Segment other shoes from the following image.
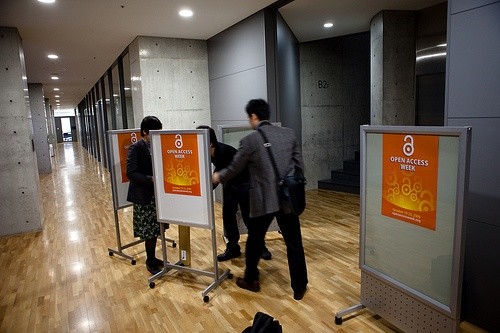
[155,257,169,267]
[145,259,162,275]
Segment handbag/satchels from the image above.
[276,175,307,217]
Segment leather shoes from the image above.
[236,277,258,291]
[262,246,272,260]
[294,288,307,300]
[217,249,241,262]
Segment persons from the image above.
[211,99,309,300]
[126,116,169,276]
[196,125,271,262]
